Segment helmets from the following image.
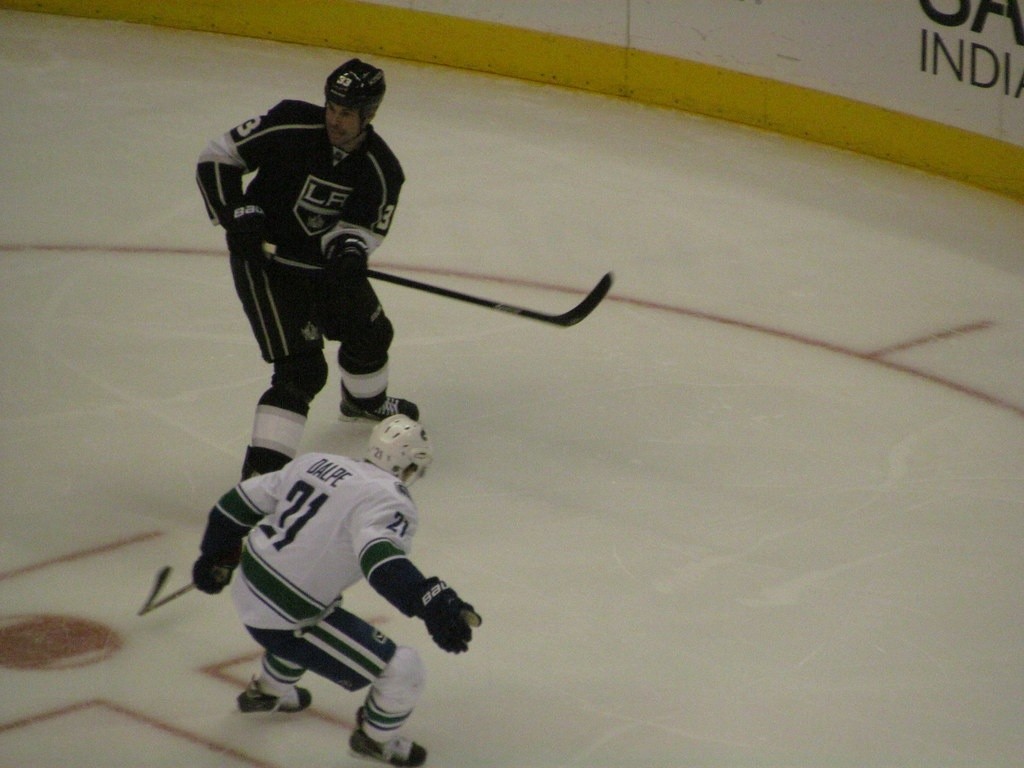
[366,414,434,487]
[325,59,386,108]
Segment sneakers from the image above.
[338,377,419,423]
[349,706,426,768]
[236,672,312,720]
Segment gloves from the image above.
[318,235,369,293]
[412,576,481,654]
[193,539,242,594]
[220,194,277,265]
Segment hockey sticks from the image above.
[139,566,198,617]
[271,247,613,328]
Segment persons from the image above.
[192,415,481,767]
[197,57,419,480]
